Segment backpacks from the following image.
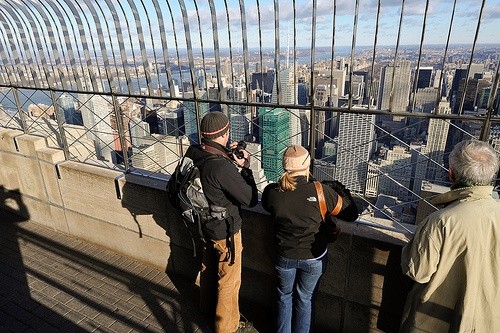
[173,154,232,225]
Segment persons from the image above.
[400,140,500,333]
[261,144,358,333]
[185,110,258,333]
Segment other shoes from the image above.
[236,320,253,333]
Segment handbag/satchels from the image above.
[313,180,342,243]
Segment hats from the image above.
[283,145,311,171]
[201,112,230,138]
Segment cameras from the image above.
[229,141,246,160]
